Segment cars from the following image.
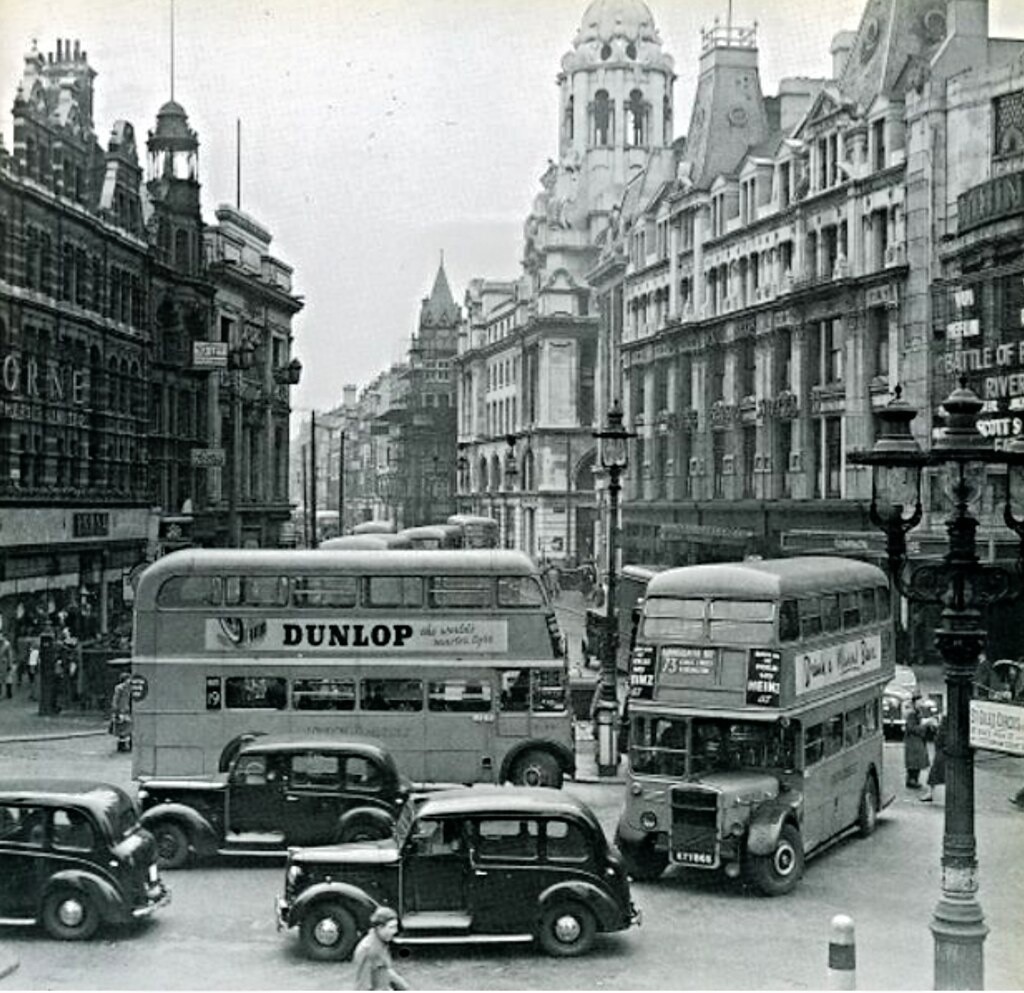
[883,685,938,741]
[130,735,464,869]
[0,776,168,941]
[274,785,642,957]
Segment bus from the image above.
[318,515,501,552]
[614,556,897,896]
[130,550,576,816]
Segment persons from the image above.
[350,907,408,991]
[113,673,131,753]
[904,695,949,803]
[0,597,99,700]
[543,557,604,608]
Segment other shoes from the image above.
[906,782,923,788]
[921,795,932,802]
[1009,796,1024,807]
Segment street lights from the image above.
[845,383,1024,991]
[593,399,639,778]
